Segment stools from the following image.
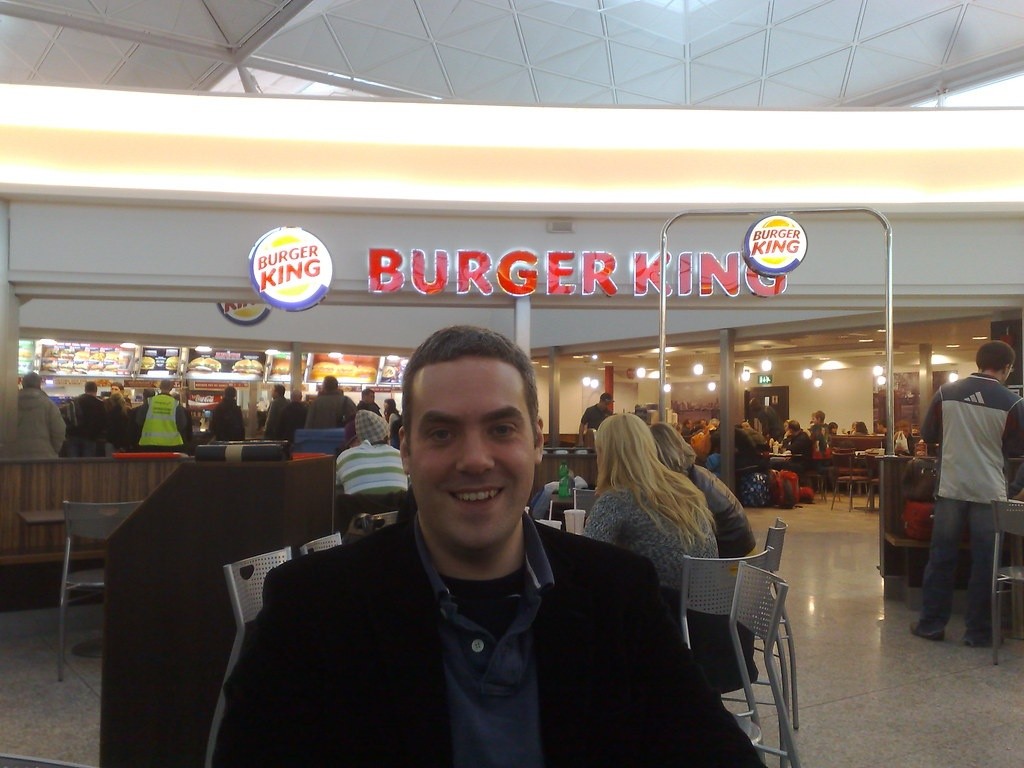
[805,472,827,502]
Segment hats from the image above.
[343,414,356,448]
[355,410,389,444]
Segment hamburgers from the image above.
[166,356,178,371]
[312,362,376,381]
[189,358,263,374]
[141,357,155,370]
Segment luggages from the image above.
[739,467,800,508]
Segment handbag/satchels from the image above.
[899,457,971,542]
[799,486,814,504]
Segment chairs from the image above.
[986,499,1024,665]
[59,450,800,768]
[830,448,882,512]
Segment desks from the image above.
[768,453,802,456]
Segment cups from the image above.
[564,509,586,535]
[877,449,885,456]
[536,519,562,530]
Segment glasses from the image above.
[1008,366,1016,373]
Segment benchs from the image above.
[16,509,118,553]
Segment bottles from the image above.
[559,460,575,498]
[769,437,783,455]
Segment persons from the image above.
[807,410,839,492]
[213,321,772,768]
[384,397,399,421]
[780,419,811,477]
[258,396,266,425]
[679,416,722,477]
[279,389,308,457]
[264,384,290,440]
[876,417,887,450]
[63,381,133,458]
[579,394,616,448]
[648,418,758,662]
[910,341,1024,648]
[209,387,245,441]
[846,421,869,435]
[356,389,383,418]
[16,372,66,458]
[584,412,731,674]
[896,419,921,457]
[734,417,776,473]
[304,375,358,431]
[131,380,192,453]
[334,412,409,494]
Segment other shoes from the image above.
[962,628,994,647]
[910,621,944,640]
